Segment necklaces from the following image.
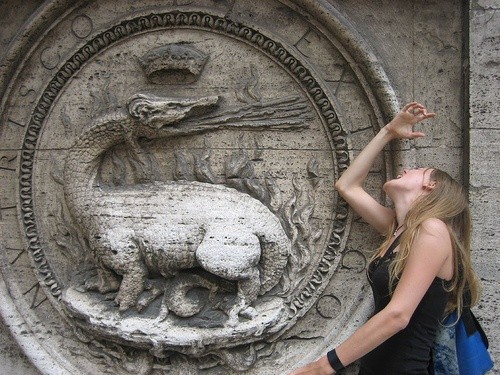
[392,223,407,237]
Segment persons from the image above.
[288,100,480,375]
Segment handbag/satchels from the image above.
[428,306,493,374]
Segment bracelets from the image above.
[326,349,347,375]
